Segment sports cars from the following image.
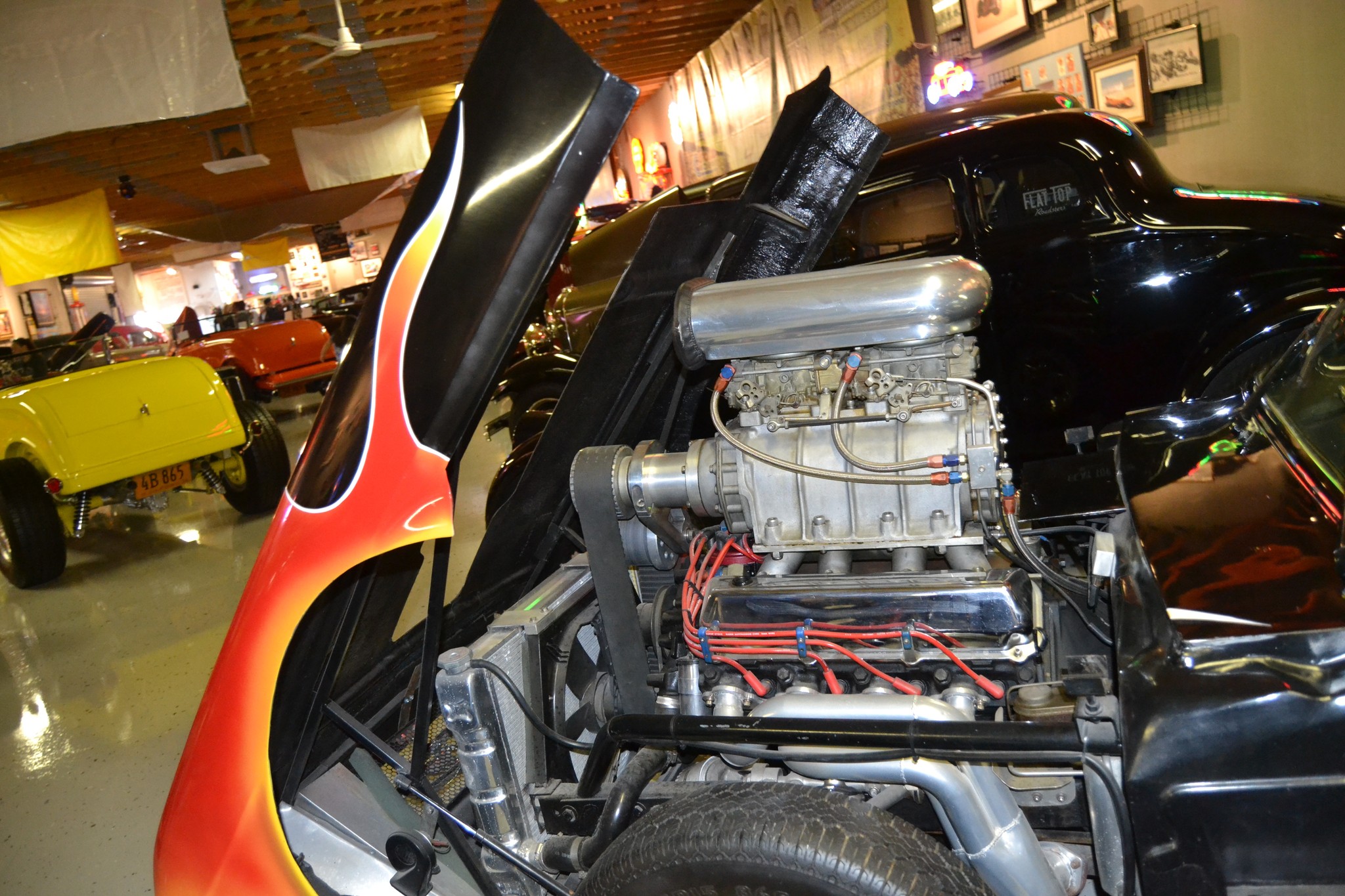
[25,324,170,372]
[93,307,341,403]
[152,0,1345,896]
[311,281,381,349]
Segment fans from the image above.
[288,0,438,71]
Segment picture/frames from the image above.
[1141,23,1208,96]
[961,0,1034,57]
[1085,1,1121,51]
[930,0,964,37]
[1085,43,1157,127]
[1018,42,1092,109]
[1028,0,1060,16]
[982,81,1026,100]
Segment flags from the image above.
[0,188,124,289]
[0,0,247,150]
[240,237,290,273]
[291,105,431,193]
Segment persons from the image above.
[2,335,48,388]
[211,286,333,333]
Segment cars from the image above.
[0,328,289,587]
